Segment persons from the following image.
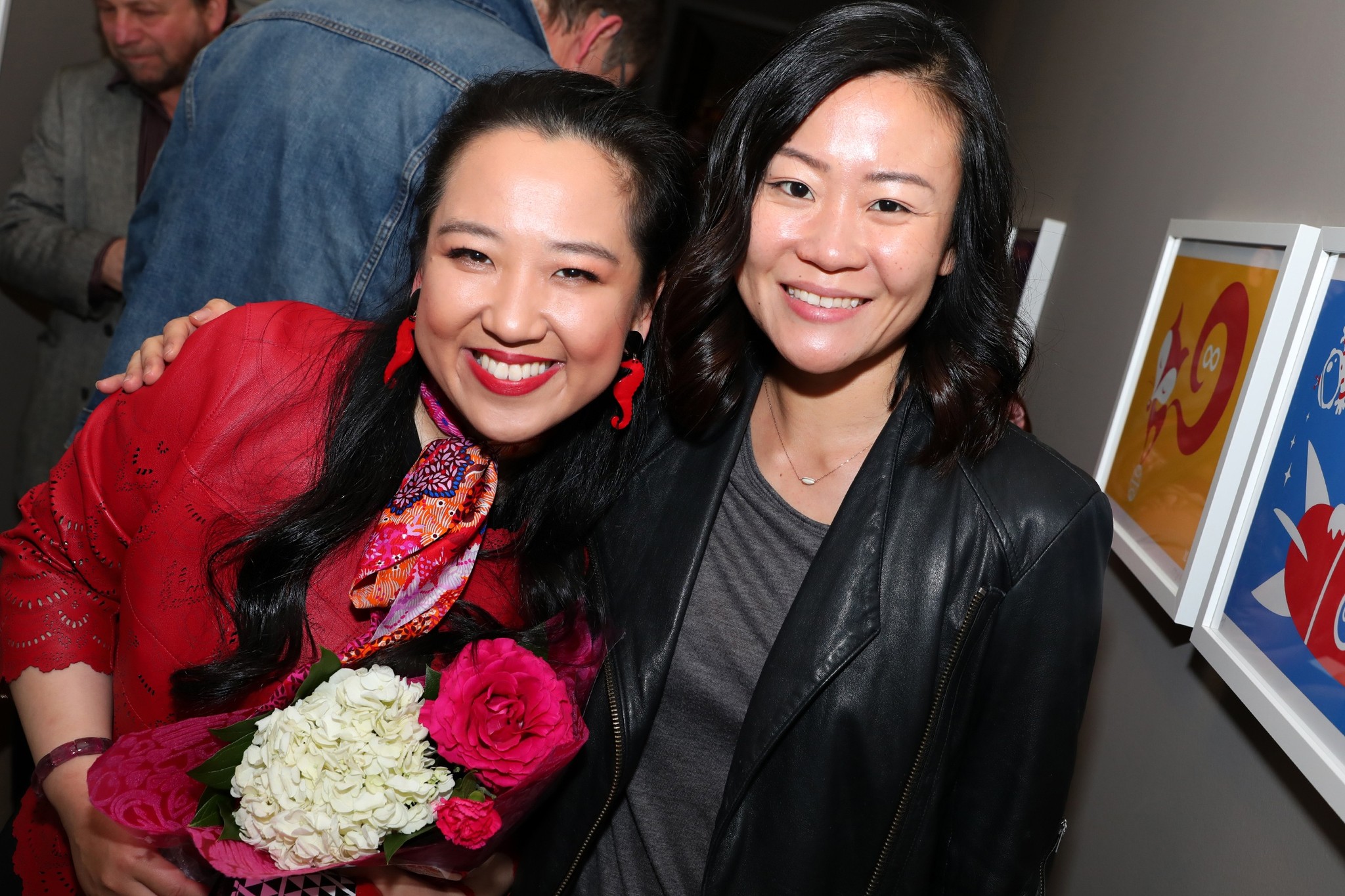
[0,0,230,533]
[95,0,1112,895]
[0,68,696,896]
[75,0,673,429]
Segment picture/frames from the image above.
[1091,216,1323,629]
[1187,224,1345,828]
[1002,215,1067,375]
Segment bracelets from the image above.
[30,737,113,800]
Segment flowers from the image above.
[84,608,607,880]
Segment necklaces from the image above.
[764,376,873,486]
